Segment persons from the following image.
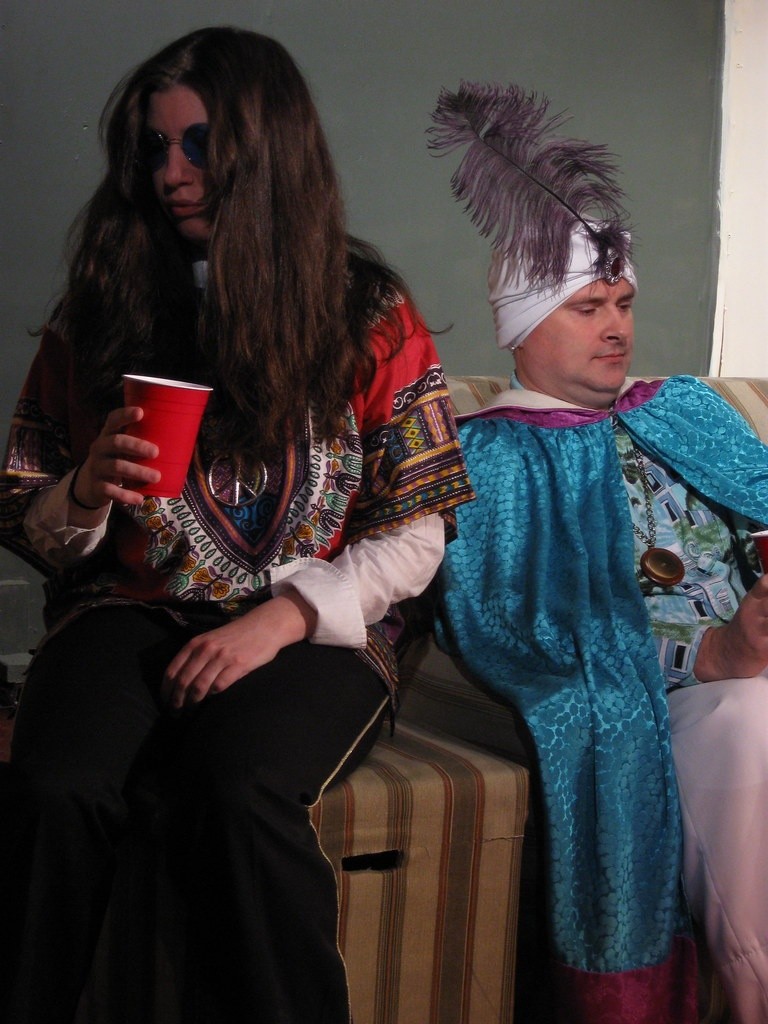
[432,206,767,1024]
[0,27,476,1024]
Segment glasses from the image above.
[133,120,222,175]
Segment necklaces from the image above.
[632,444,685,584]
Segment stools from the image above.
[0,711,530,1024]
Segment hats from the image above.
[422,78,639,353]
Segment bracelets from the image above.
[70,461,110,510]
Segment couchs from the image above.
[397,375,767,1024]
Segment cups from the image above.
[751,530,768,574]
[121,374,213,503]
